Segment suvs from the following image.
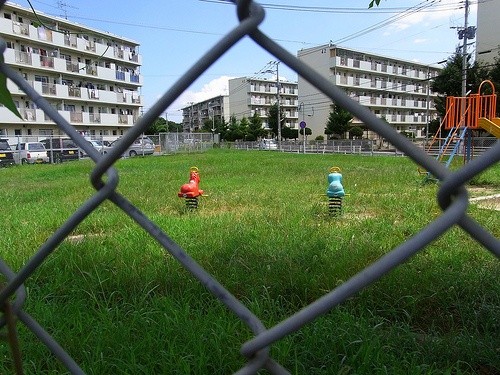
[0,138,16,167]
[39,138,79,163]
[110,135,155,157]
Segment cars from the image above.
[81,140,113,158]
[256,139,278,150]
[10,143,49,166]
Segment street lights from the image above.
[299,101,305,154]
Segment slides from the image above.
[478,117,500,139]
